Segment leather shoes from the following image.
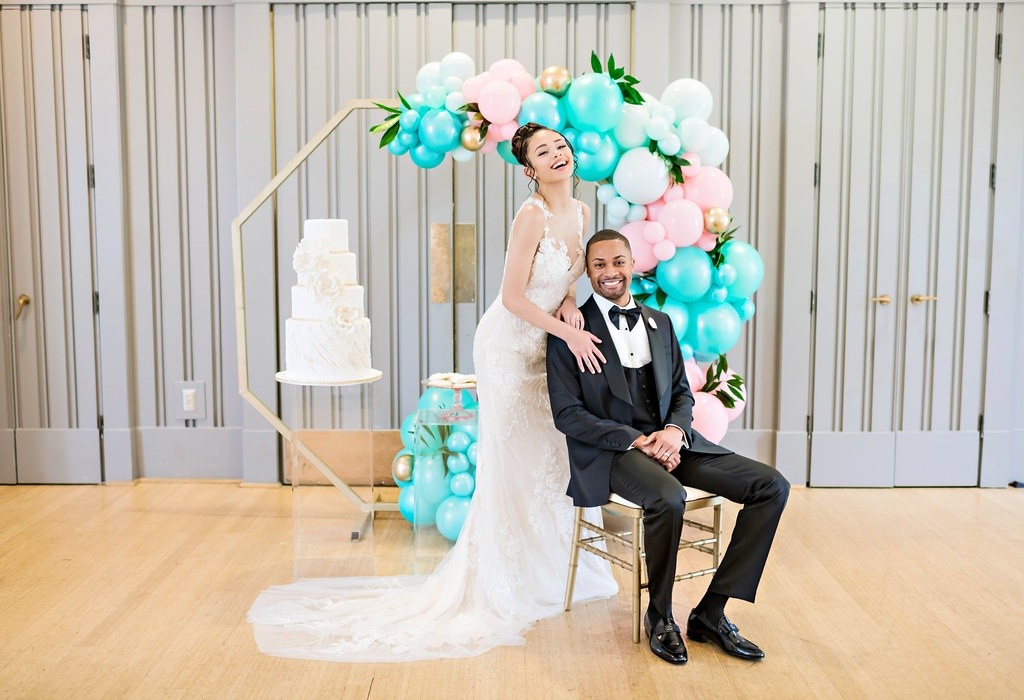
[687,607,764,659]
[644,609,688,663]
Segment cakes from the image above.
[285,219,371,382]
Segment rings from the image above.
[576,320,579,321]
[665,454,669,457]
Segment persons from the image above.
[454,121,620,614]
[546,229,792,664]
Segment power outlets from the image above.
[182,389,196,411]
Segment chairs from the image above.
[563,482,727,643]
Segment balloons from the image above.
[391,387,479,541]
[625,153,763,444]
[540,67,572,97]
[479,59,536,155]
[704,207,729,233]
[497,71,627,183]
[597,80,729,227]
[387,52,485,168]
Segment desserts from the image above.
[428,372,475,389]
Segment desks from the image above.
[274,368,384,588]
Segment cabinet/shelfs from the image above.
[411,407,481,572]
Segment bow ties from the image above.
[608,305,642,331]
